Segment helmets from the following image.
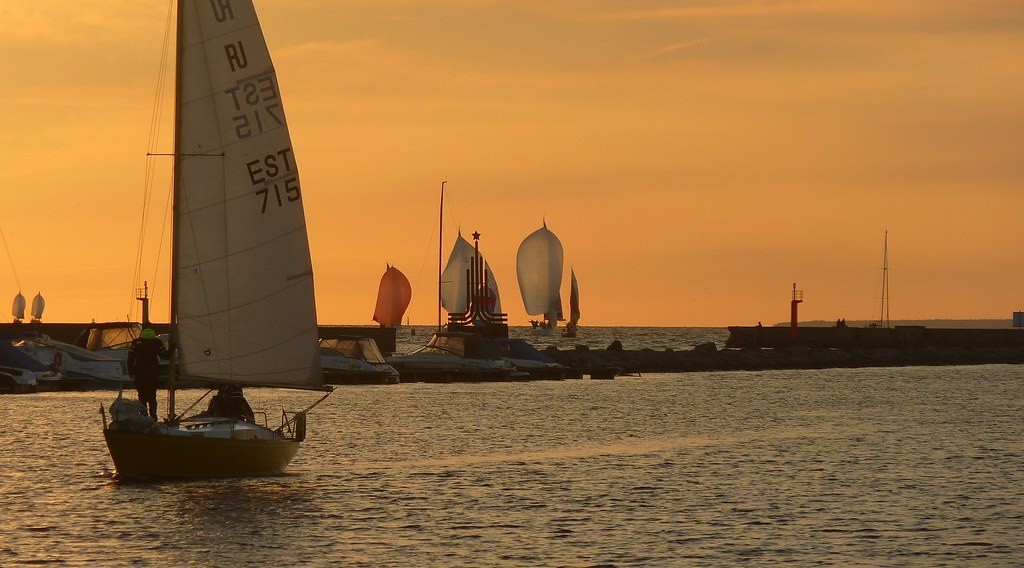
[140,328,155,340]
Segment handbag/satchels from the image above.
[128,347,148,378]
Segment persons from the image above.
[127,328,169,422]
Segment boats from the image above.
[1,321,622,394]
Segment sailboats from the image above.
[423,178,509,347]
[12,290,26,323]
[561,270,580,339]
[516,218,567,337]
[29,290,46,324]
[371,261,412,327]
[99,1,339,482]
